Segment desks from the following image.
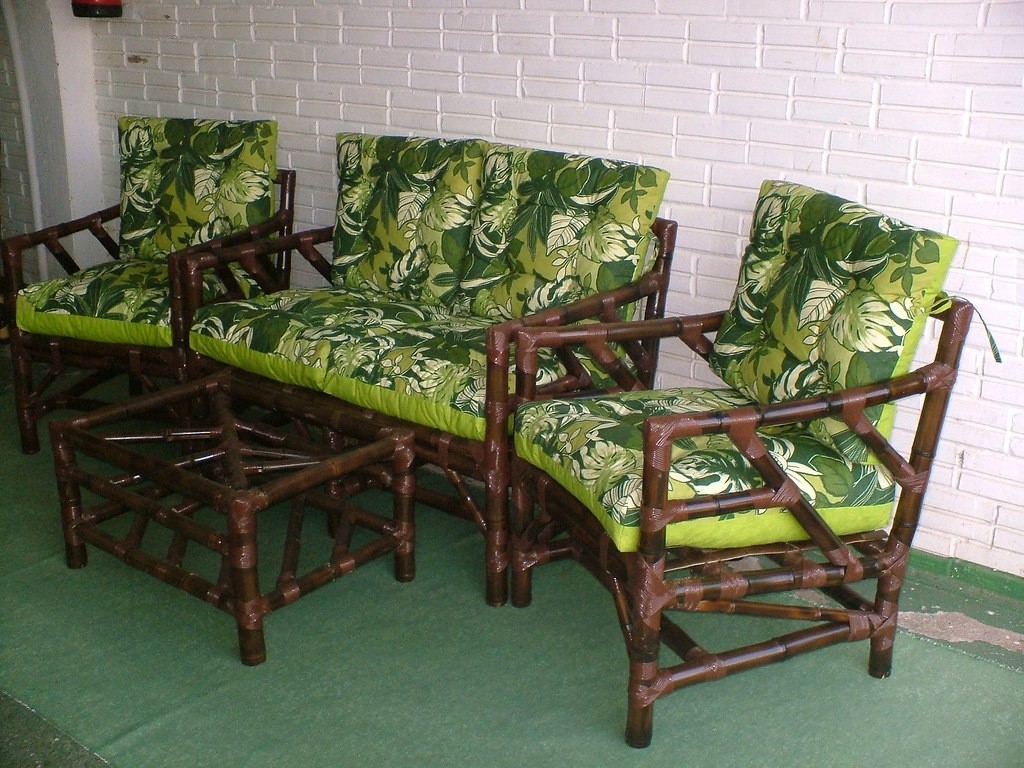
[48,370,416,668]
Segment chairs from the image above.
[0,115,298,482]
[509,179,1005,749]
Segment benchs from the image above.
[178,131,679,608]
